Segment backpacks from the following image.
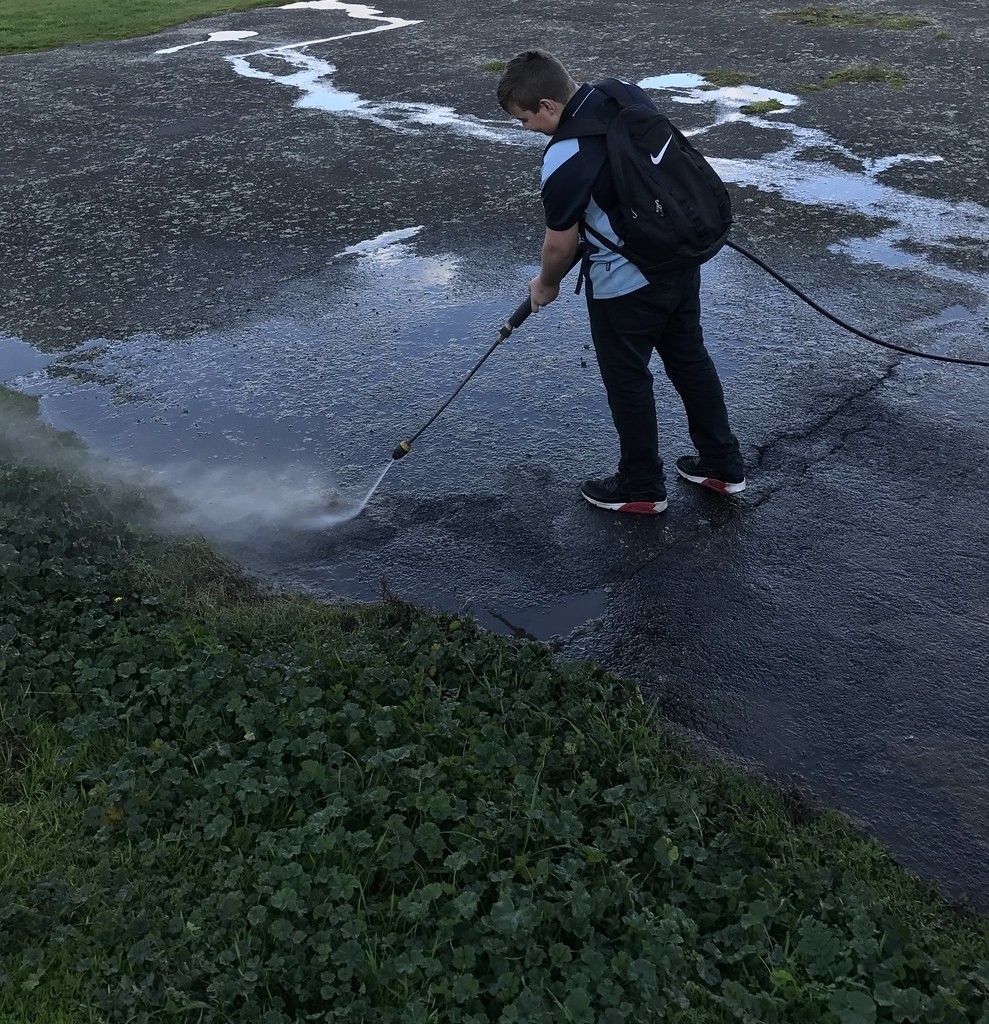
[541,77,732,272]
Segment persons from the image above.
[494,49,748,513]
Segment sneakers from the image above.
[676,455,746,495]
[580,472,668,515]
